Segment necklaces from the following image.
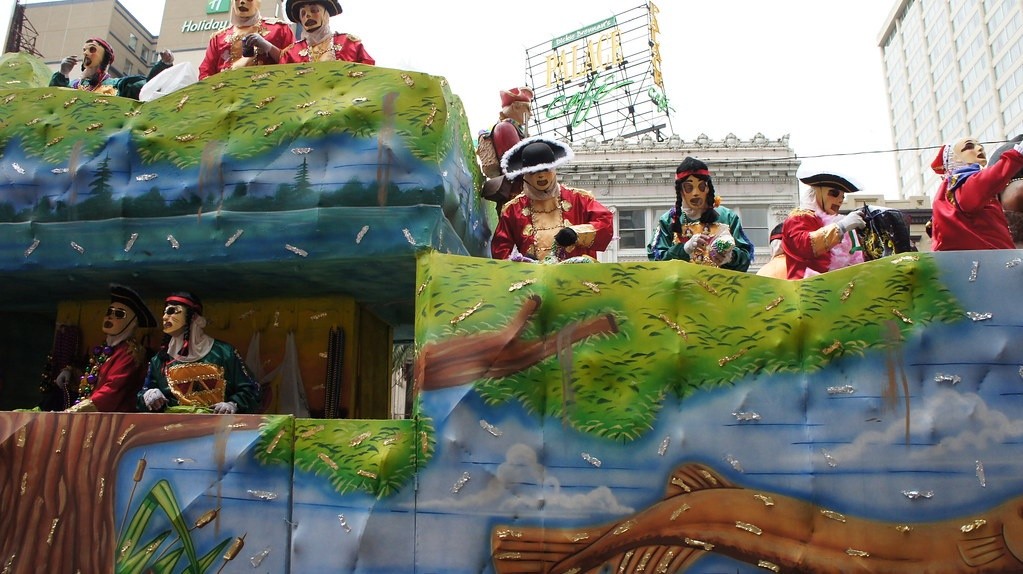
[325,326,344,418]
[75,343,112,404]
[64,384,70,409]
[91,73,108,91]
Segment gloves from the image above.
[840,210,866,232]
[55,366,74,388]
[61,55,78,75]
[555,227,577,248]
[245,33,272,54]
[209,401,238,415]
[142,388,168,411]
[160,49,175,64]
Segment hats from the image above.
[285,0,343,23]
[86,38,115,65]
[932,143,949,175]
[796,157,864,193]
[500,137,574,179]
[109,283,160,328]
[500,86,534,107]
[165,292,204,316]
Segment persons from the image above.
[755,223,785,279]
[647,156,752,272]
[136,291,262,414]
[493,87,534,205]
[492,136,613,261]
[930,135,1023,252]
[280,0,375,65]
[199,0,296,79]
[783,173,867,279]
[49,38,174,99]
[54,284,157,411]
